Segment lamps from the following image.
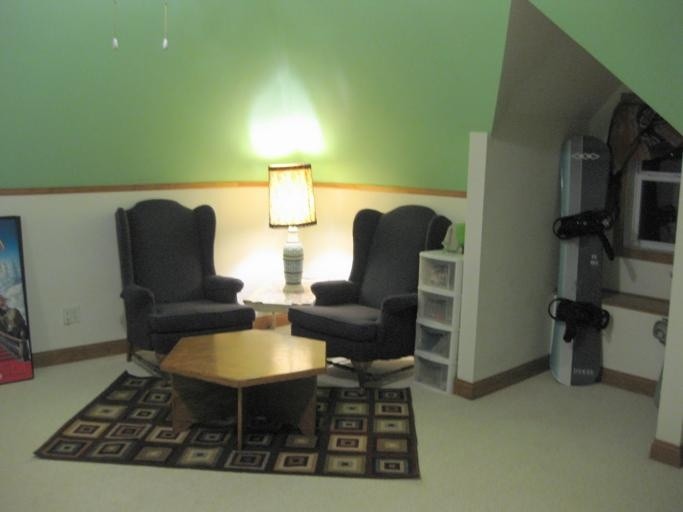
[266,161,319,292]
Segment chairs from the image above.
[288,203,452,398]
[115,198,256,368]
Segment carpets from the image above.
[32,366,425,482]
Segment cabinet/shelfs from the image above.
[413,246,455,397]
